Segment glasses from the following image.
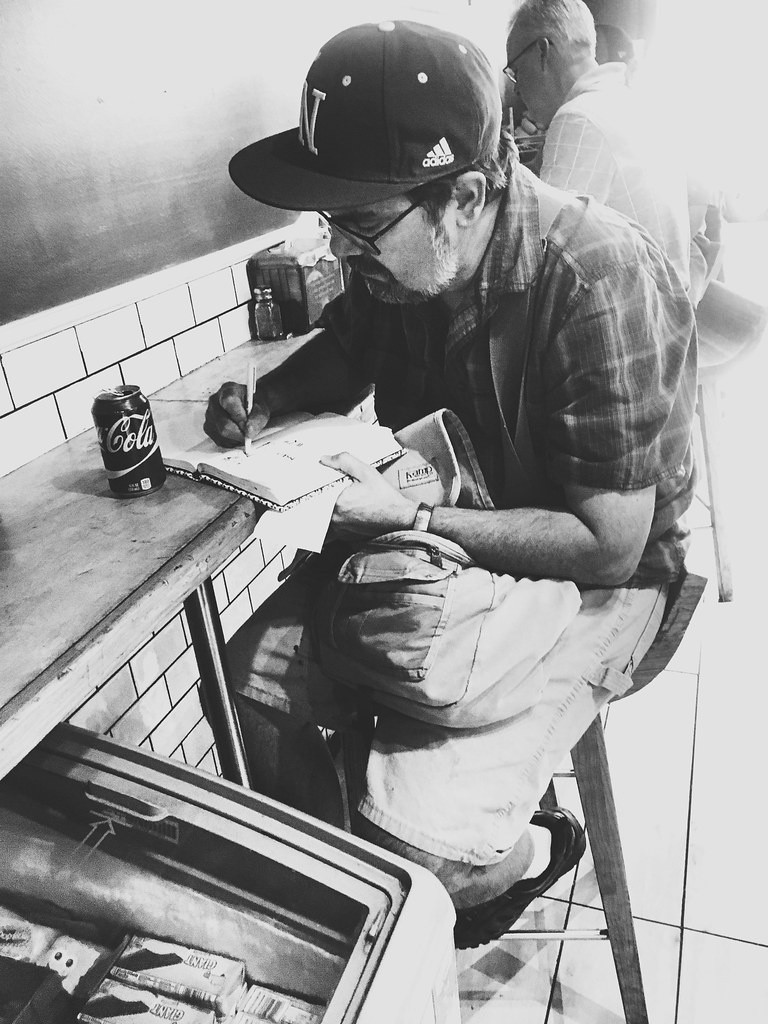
[314,187,449,258]
[502,36,553,84]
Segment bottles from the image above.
[253,285,282,341]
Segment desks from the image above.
[0,328,376,789]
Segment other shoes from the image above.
[454,806,587,951]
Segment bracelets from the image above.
[412,500,434,533]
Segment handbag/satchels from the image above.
[306,526,582,730]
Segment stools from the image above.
[689,281,768,602]
[343,573,708,1024]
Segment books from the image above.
[162,409,409,512]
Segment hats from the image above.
[227,19,502,211]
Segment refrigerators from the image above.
[0,720,460,1024]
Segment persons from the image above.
[499,0,722,315]
[202,22,699,949]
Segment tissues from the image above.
[246,210,345,335]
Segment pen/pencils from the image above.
[244,363,257,457]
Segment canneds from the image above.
[90,385,166,498]
